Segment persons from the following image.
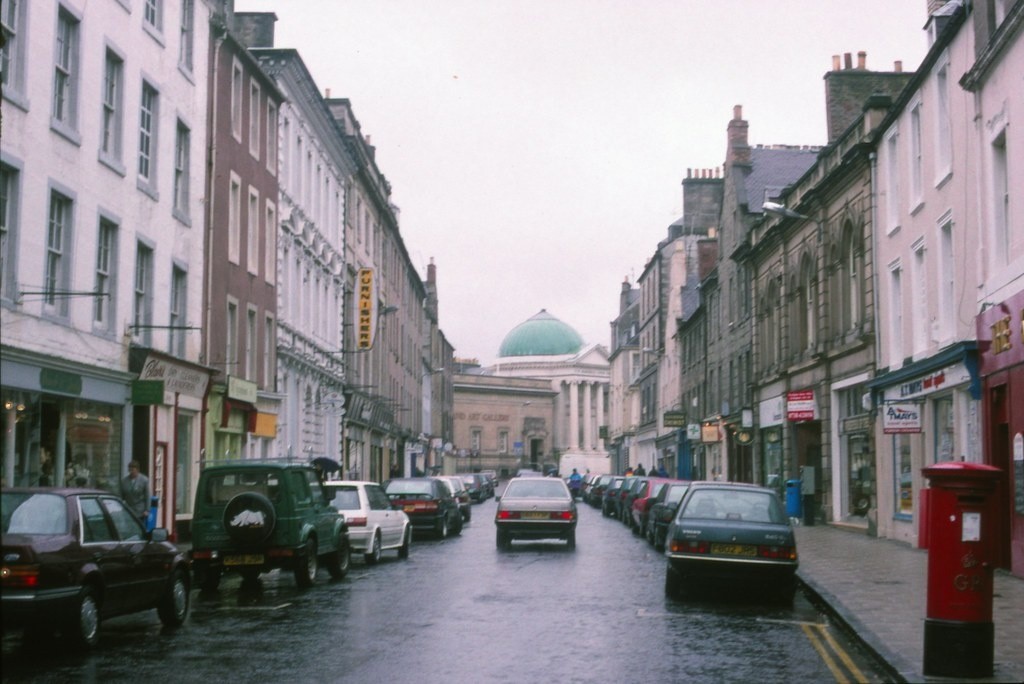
[621,462,669,478]
[39,449,89,489]
[390,463,444,478]
[316,463,327,481]
[120,460,151,528]
[569,469,581,502]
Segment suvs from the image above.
[193,459,352,591]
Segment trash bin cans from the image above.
[784,479,802,518]
[144,496,159,534]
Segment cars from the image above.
[580,473,691,552]
[386,477,463,540]
[444,470,499,521]
[494,476,577,547]
[515,468,545,477]
[1,487,194,654]
[665,480,798,586]
[309,480,413,566]
